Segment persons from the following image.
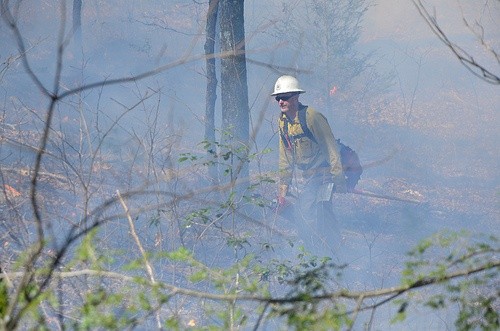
[269,74,361,259]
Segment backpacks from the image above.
[283,105,362,194]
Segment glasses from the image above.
[274,93,297,101]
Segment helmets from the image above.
[270,75,306,96]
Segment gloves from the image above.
[278,185,289,205]
[334,174,347,196]
